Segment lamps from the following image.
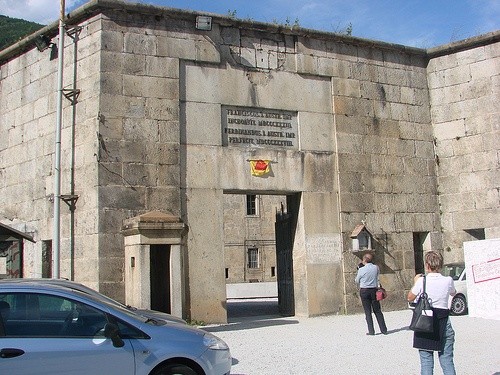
[34,35,51,51]
[195,15,212,31]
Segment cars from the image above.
[0,277,232,375]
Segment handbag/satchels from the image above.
[409,292,434,334]
[376,288,387,301]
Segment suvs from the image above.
[408,262,468,316]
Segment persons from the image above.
[355,254,387,335]
[408,251,456,375]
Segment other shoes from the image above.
[366,333,375,336]
